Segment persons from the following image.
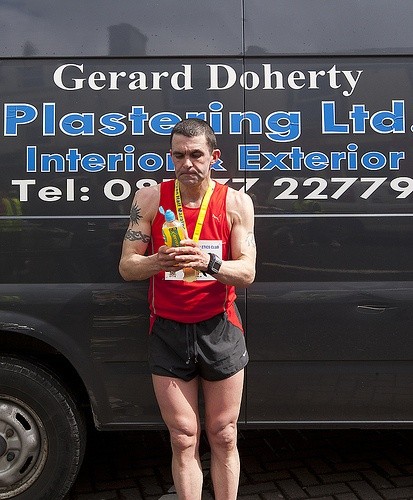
[119,119,256,499]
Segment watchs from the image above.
[200,253,221,277]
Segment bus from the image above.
[0,1,413,500]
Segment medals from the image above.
[181,263,197,284]
